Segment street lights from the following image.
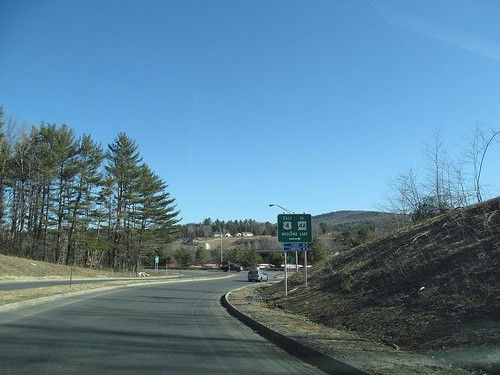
[268,203,298,275]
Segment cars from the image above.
[248,270,269,282]
[222,263,244,272]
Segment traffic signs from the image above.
[278,213,313,243]
